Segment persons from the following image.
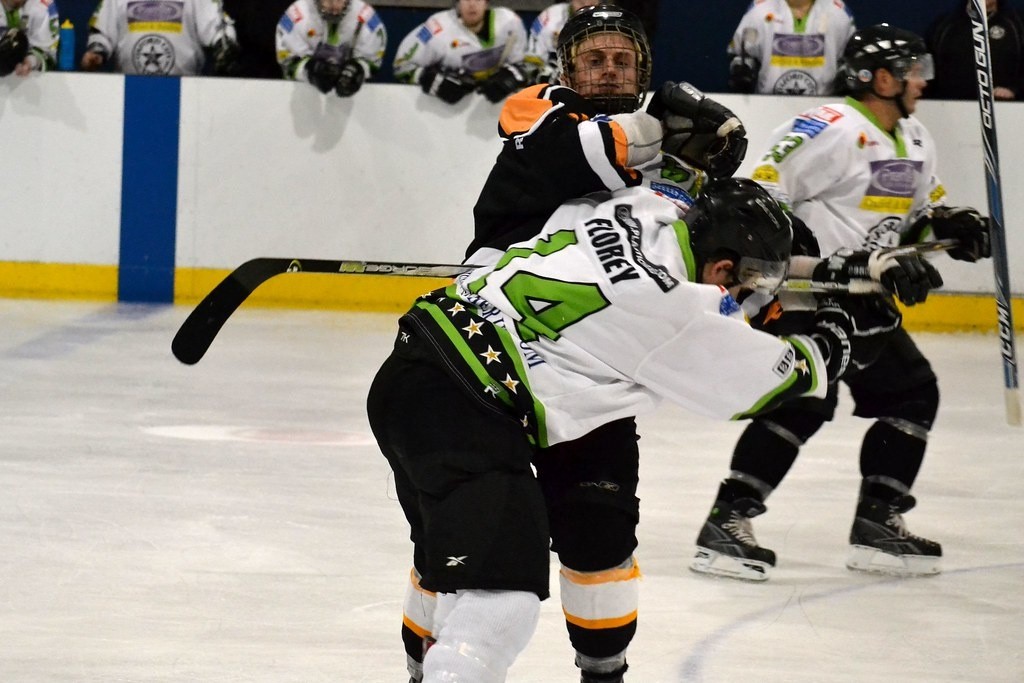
[730,0,860,98]
[684,21,992,583]
[0,0,241,80]
[276,0,387,97]
[367,6,898,682]
[395,0,605,107]
[933,1,1018,100]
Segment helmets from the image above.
[842,24,927,90]
[690,178,793,295]
[557,6,652,73]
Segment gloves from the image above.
[931,206,992,263]
[418,62,478,105]
[309,58,365,97]
[480,61,529,104]
[807,247,944,385]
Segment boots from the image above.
[847,493,944,573]
[689,482,776,582]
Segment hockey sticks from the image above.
[169,256,884,369]
[886,239,962,260]
[970,0,1023,427]
